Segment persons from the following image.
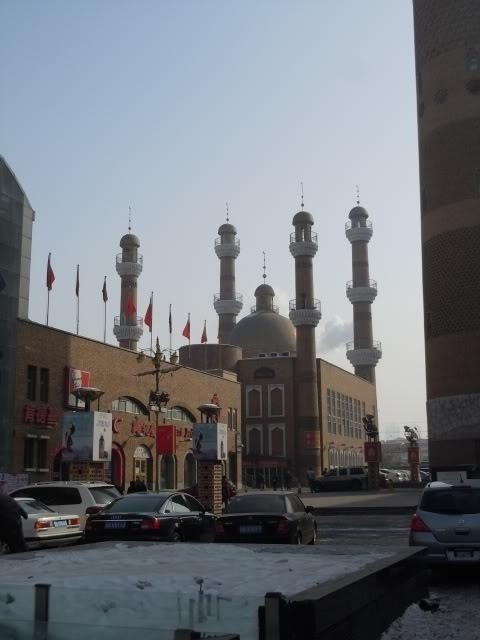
[211,392,220,423]
[136,477,148,492]
[126,481,137,494]
[284,470,293,491]
[222,475,229,510]
[306,467,315,493]
[321,467,328,475]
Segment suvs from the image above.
[406,475,479,567]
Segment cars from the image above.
[6,479,214,546]
[211,490,319,547]
[311,464,431,491]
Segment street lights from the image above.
[136,336,183,491]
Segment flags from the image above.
[102,279,108,303]
[143,298,152,332]
[182,320,189,338]
[200,322,207,343]
[77,266,80,297]
[169,306,172,333]
[47,256,55,291]
